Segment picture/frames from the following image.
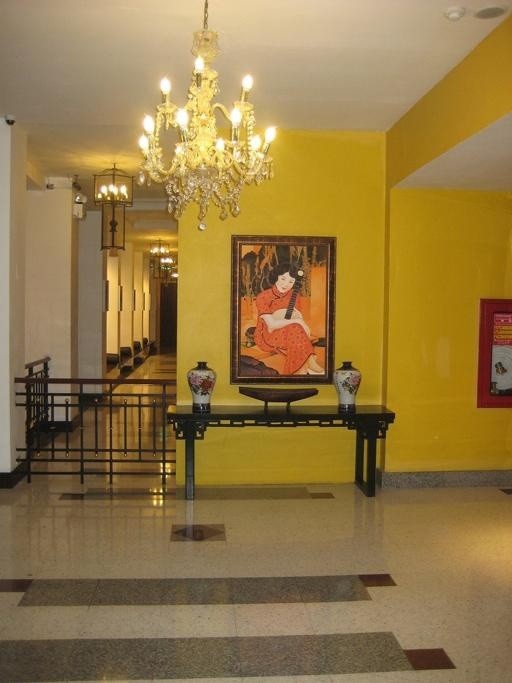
[225,231,335,385]
[476,297,511,409]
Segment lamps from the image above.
[137,1,277,230]
[147,238,178,280]
[93,162,134,258]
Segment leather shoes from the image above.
[308,368,324,375]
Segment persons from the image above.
[254,261,325,375]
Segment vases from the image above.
[186,359,216,411]
[331,360,360,412]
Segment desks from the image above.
[164,403,394,501]
